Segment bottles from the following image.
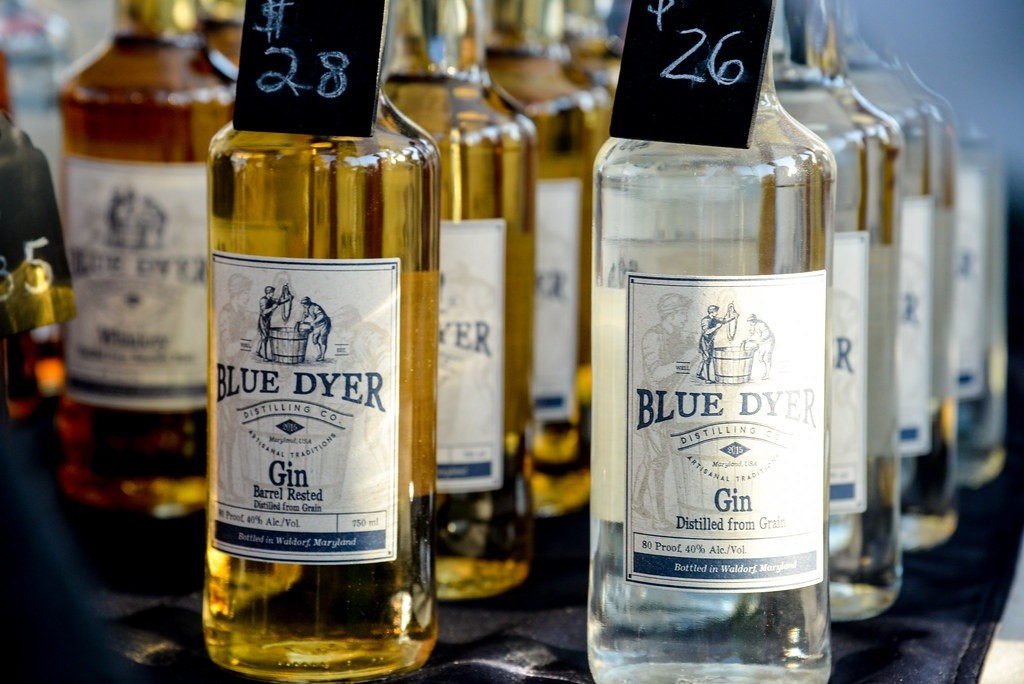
[1,0,1007,684]
